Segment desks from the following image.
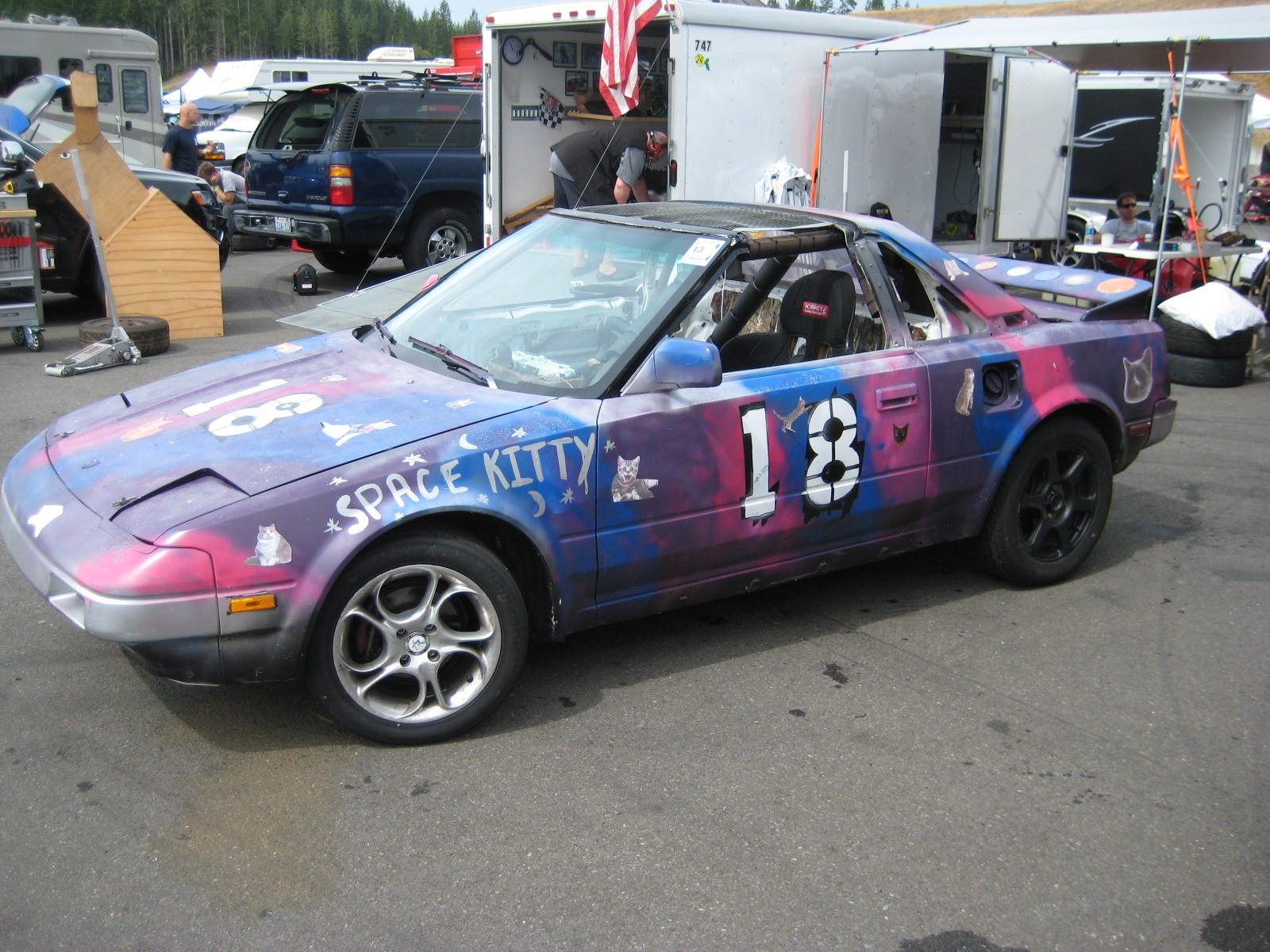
[1074,240,1262,292]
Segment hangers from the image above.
[516,35,554,62]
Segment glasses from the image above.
[1120,202,1136,208]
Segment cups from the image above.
[207,139,214,154]
[1101,233,1114,249]
[1144,234,1153,242]
[1177,243,1191,251]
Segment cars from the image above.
[0,199,1180,748]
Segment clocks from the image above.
[501,35,524,64]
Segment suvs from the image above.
[0,104,231,302]
[194,101,275,178]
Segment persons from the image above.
[162,103,215,177]
[1094,192,1154,279]
[549,128,668,282]
[197,161,247,253]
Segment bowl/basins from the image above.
[1243,238,1255,246]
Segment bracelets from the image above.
[212,186,220,191]
[203,148,206,155]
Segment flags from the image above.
[599,0,664,118]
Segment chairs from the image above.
[719,270,856,372]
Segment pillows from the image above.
[1156,281,1268,340]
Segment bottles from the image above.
[1083,217,1095,247]
[1197,228,1207,242]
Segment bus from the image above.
[1,21,169,171]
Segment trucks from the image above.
[481,0,1257,249]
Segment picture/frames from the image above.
[646,74,666,90]
[660,47,669,73]
[639,46,656,72]
[565,70,588,95]
[592,71,600,93]
[553,41,577,68]
[581,43,603,69]
